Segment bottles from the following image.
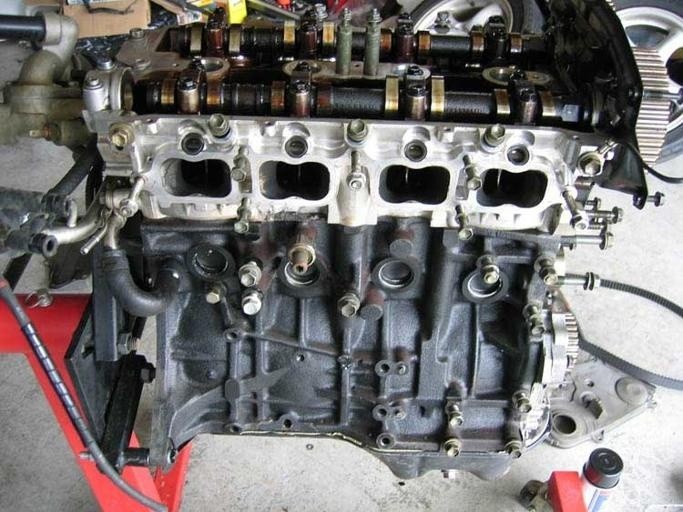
[580,447,624,512]
